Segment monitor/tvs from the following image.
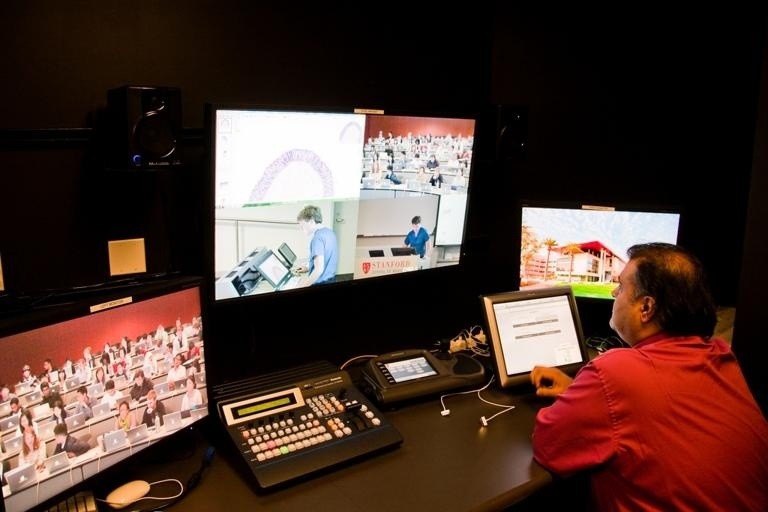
[278,243,296,266]
[0,275,216,512]
[391,247,414,255]
[368,249,384,257]
[254,250,289,289]
[206,102,482,308]
[513,194,683,315]
[479,292,594,394]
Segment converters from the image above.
[450,335,486,351]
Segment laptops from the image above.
[363,138,468,193]
[1,347,204,492]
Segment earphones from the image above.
[480,416,488,427]
[440,409,451,417]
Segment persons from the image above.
[0,310,206,480]
[362,125,473,194]
[403,214,431,260]
[530,240,767,512]
[287,204,339,288]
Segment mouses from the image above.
[104,478,151,511]
